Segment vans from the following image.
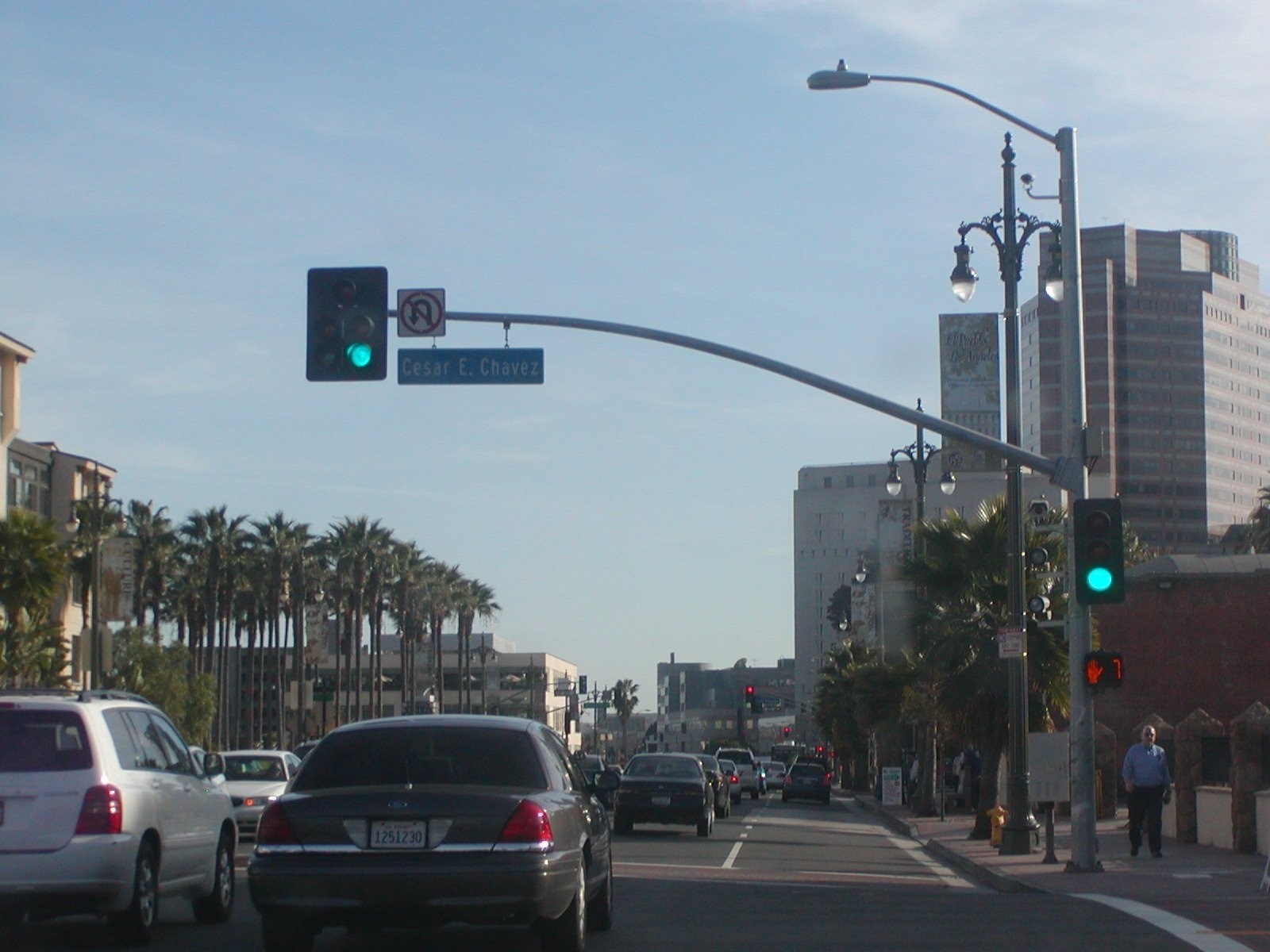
[794,755,828,773]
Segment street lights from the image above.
[278,549,327,745]
[947,129,1064,852]
[396,609,428,716]
[521,653,545,721]
[885,397,957,816]
[65,461,127,690]
[854,550,890,802]
[808,57,1100,873]
[468,632,497,714]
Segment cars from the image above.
[572,754,604,785]
[759,761,788,795]
[187,740,321,839]
[245,712,622,952]
[719,759,744,806]
[613,751,718,837]
[655,753,733,820]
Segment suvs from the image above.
[715,747,761,800]
[782,764,831,805]
[0,686,239,950]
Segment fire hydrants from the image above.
[986,804,1008,848]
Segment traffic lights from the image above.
[745,684,754,703]
[784,727,789,737]
[818,747,823,759]
[1085,652,1123,687]
[306,265,389,382]
[1073,497,1125,605]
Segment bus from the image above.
[771,741,807,772]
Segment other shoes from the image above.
[1148,849,1162,858]
[1130,839,1142,857]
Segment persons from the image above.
[1121,726,1171,857]
[906,748,965,807]
